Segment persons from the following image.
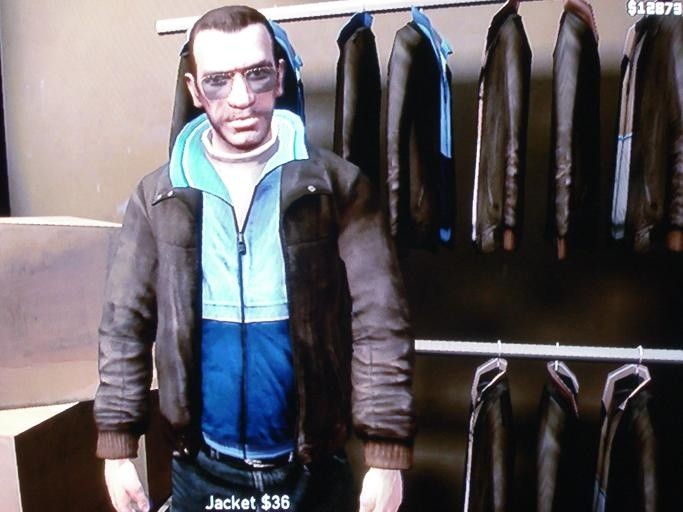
[93,5,415,512]
